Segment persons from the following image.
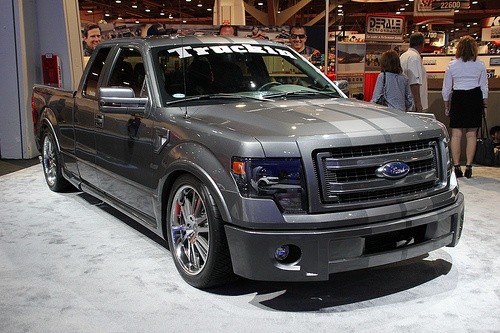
[442,35,489,178]
[399,32,429,113]
[81,22,323,64]
[367,49,413,112]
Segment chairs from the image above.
[186,59,213,97]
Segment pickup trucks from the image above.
[28,32,466,292]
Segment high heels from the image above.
[465,165,472,178]
[454,165,463,178]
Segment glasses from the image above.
[290,33,305,39]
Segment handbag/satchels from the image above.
[475,140,495,163]
[376,95,388,107]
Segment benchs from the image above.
[118,61,145,86]
